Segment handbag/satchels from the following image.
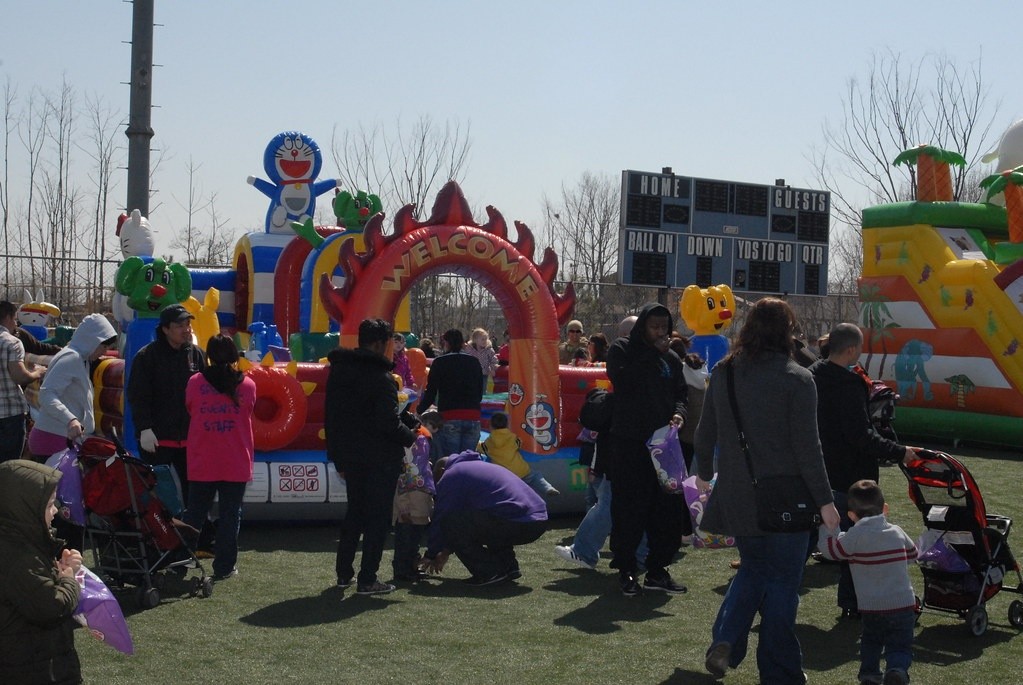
[579,390,614,432]
[681,471,738,548]
[645,423,689,495]
[43,446,88,527]
[917,529,980,591]
[71,564,135,656]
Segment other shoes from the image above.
[729,560,740,568]
[459,567,523,586]
[217,565,238,579]
[810,551,848,564]
[884,672,904,685]
[682,533,696,545]
[706,641,732,676]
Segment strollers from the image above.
[897,445,1023,637]
[853,363,898,465]
[66,425,214,608]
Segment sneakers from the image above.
[337,576,355,588]
[355,578,396,594]
[620,568,640,597]
[552,543,593,570]
[642,573,686,594]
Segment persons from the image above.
[0,459,84,685]
[0,301,256,578]
[325,297,922,685]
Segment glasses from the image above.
[569,330,580,333]
[588,342,594,345]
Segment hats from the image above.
[566,320,583,336]
[159,304,195,322]
